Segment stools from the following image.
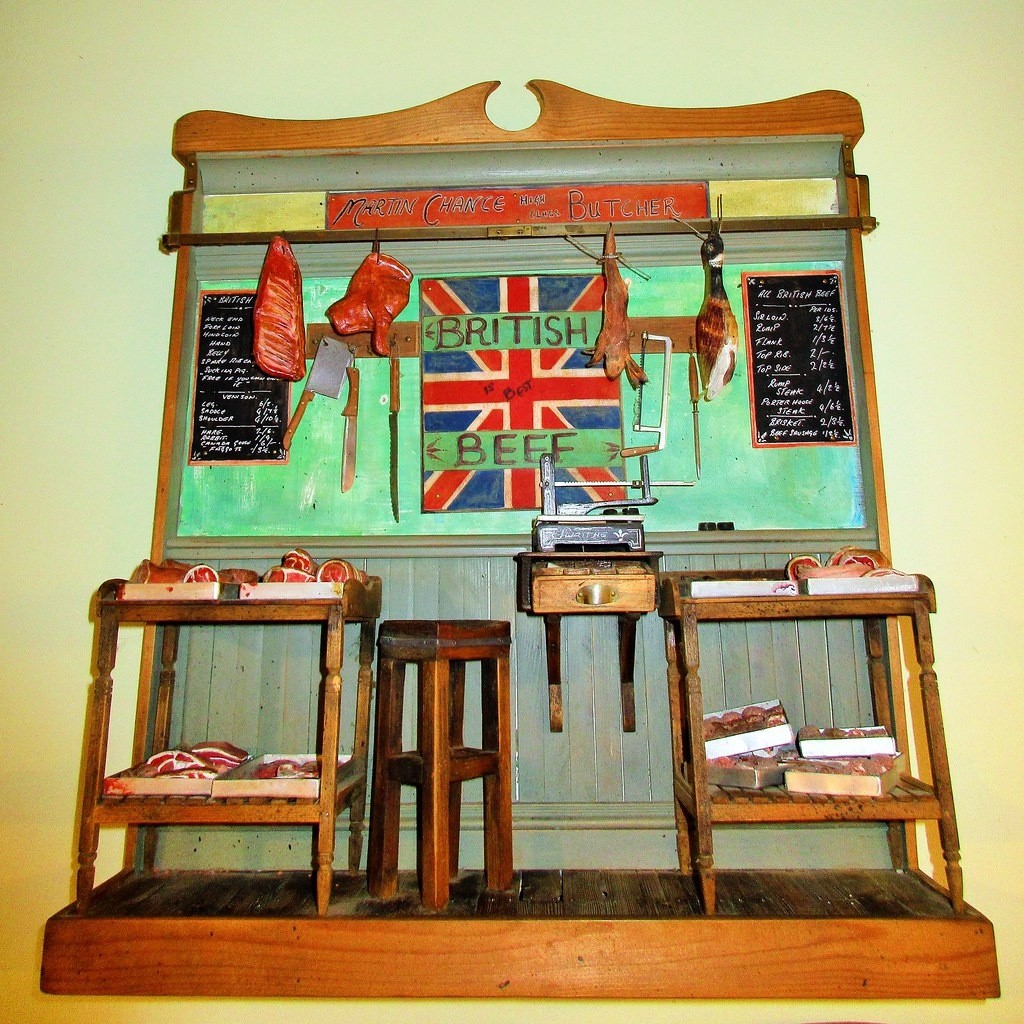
[365,617,513,908]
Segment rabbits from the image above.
[581,222,648,389]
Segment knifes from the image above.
[284,336,354,451]
[389,359,400,523]
[342,367,359,493]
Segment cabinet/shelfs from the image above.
[654,567,967,918]
[75,558,383,914]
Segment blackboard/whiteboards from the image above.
[744,272,857,445]
[187,287,290,464]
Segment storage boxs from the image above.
[703,699,906,796]
[212,754,354,800]
[97,746,254,799]
[691,575,921,599]
[118,582,345,600]
[529,563,657,616]
